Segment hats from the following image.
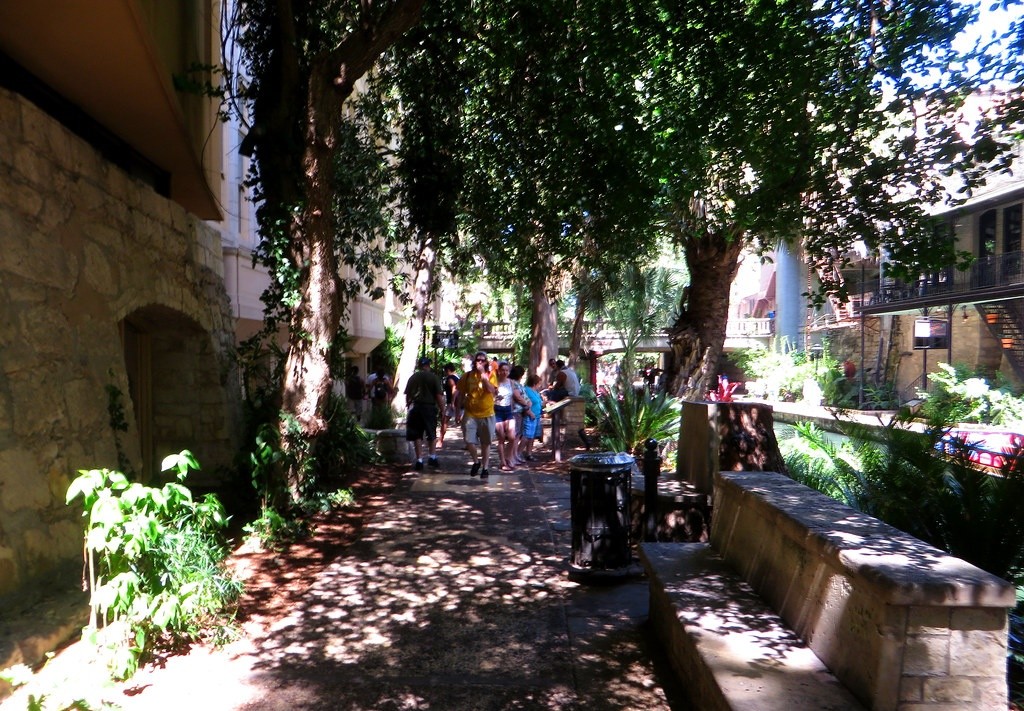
[418,357,430,364]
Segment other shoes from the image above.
[416,462,423,470]
[525,454,537,462]
[428,458,439,467]
[498,458,515,471]
[512,452,526,465]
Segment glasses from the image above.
[476,358,486,362]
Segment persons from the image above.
[595,385,608,402]
[346,365,399,423]
[640,363,663,395]
[404,351,580,480]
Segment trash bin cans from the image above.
[567,452,636,570]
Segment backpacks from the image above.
[374,385,388,399]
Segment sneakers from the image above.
[480,468,488,478]
[470,460,481,476]
